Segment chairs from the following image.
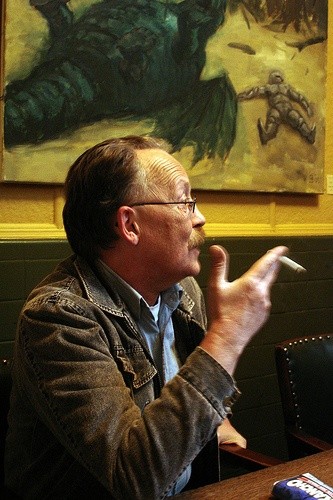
[275,333,333,461]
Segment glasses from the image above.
[114,197,196,228]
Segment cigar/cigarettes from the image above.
[267,250,307,273]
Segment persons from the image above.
[0,135,289,500]
